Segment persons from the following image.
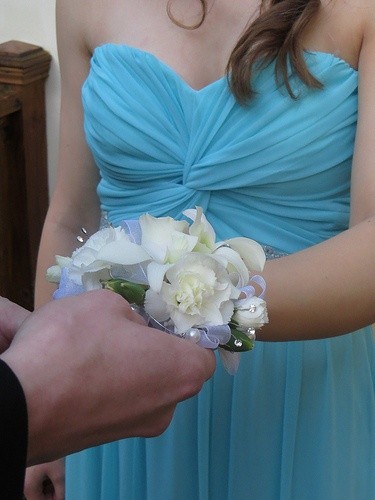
[21,1,375,500]
[0,288,215,500]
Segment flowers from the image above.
[45,209,271,377]
